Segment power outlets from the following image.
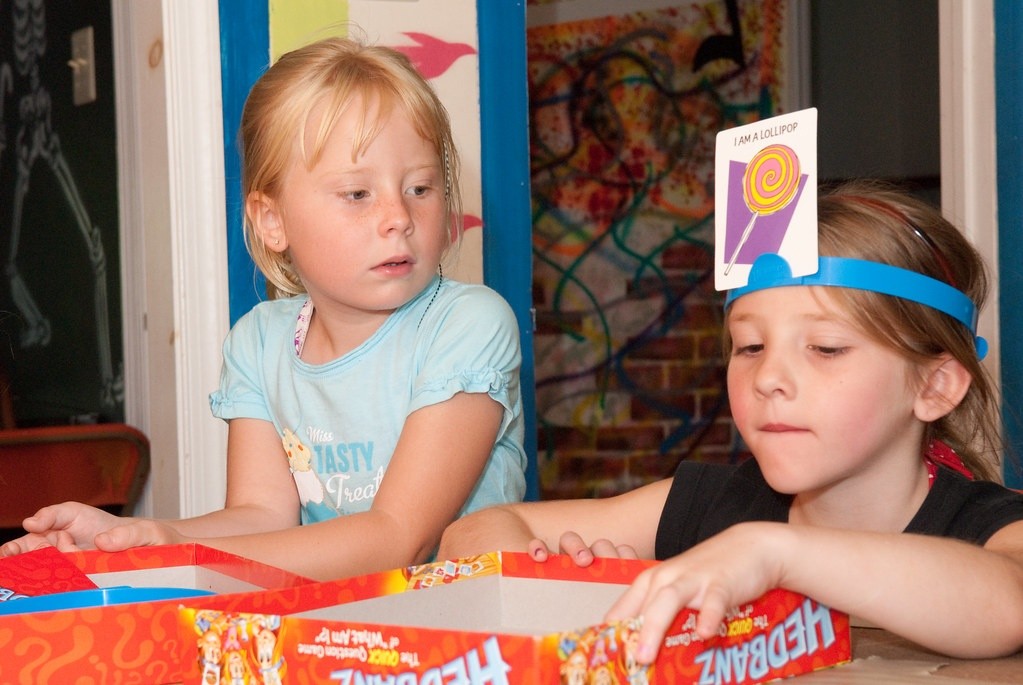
[70,25,97,105]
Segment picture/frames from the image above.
[527,0,813,247]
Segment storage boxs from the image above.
[1,541,329,685]
[171,550,852,685]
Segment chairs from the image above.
[1,425,151,534]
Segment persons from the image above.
[0,35,532,579]
[433,178,1023,665]
